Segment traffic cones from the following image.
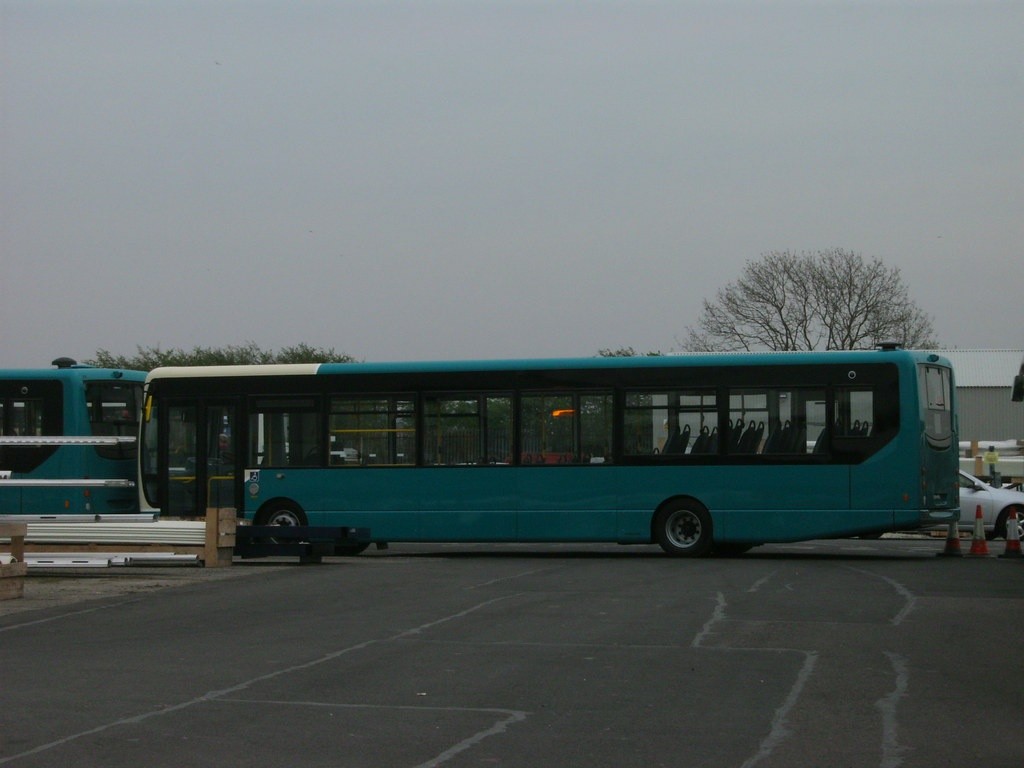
[995,504,1023,560]
[964,504,999,560]
[935,506,971,559]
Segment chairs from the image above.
[813,420,869,454]
[691,419,790,453]
[652,424,690,455]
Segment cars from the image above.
[924,466,1024,541]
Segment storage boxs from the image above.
[0,522,29,601]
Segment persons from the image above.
[216,434,234,464]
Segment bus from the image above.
[0,356,237,540]
[120,341,960,557]
